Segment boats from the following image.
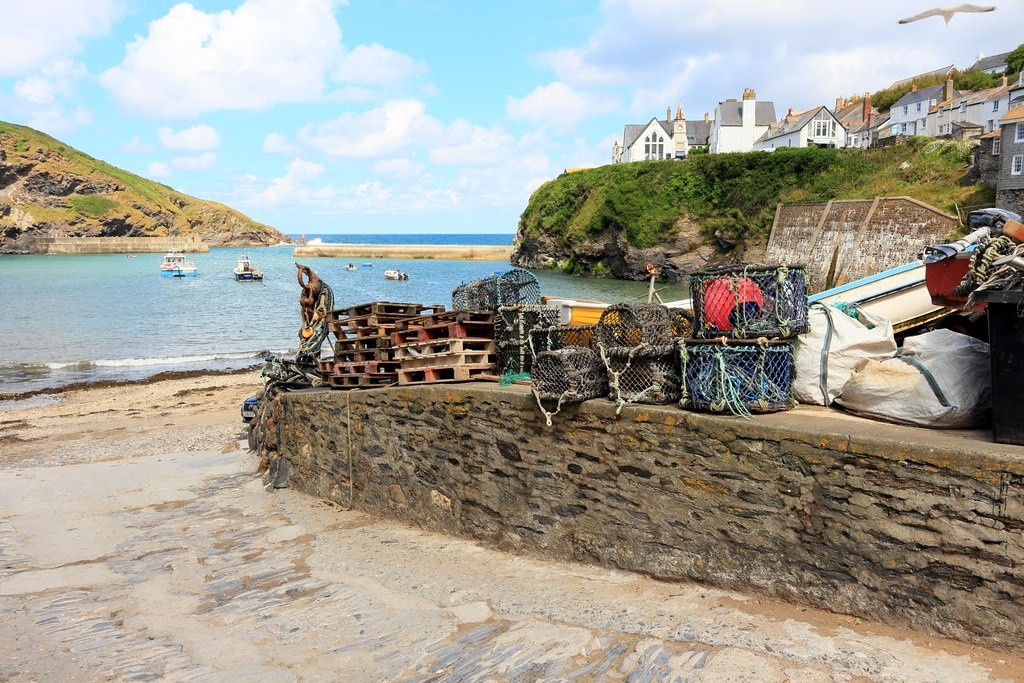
[161,248,197,279]
[807,208,1024,345]
[540,296,697,347]
[344,263,355,271]
[362,263,373,266]
[384,270,409,281]
[233,249,263,282]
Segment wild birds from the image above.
[898,4,997,25]
